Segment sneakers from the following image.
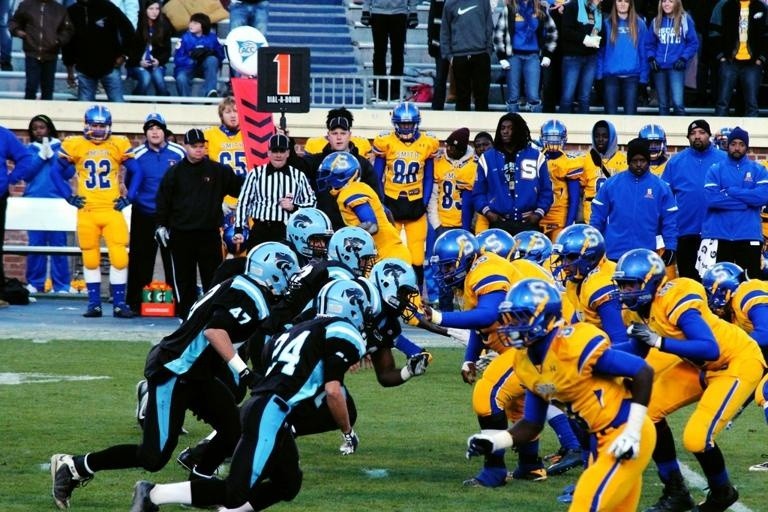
[548,450,582,475]
[188,466,223,482]
[421,351,432,363]
[83,304,102,317]
[114,303,133,318]
[544,446,568,460]
[749,461,768,472]
[205,89,217,105]
[136,379,149,428]
[643,487,695,512]
[179,426,188,434]
[50,289,68,294]
[514,466,547,481]
[50,453,86,510]
[697,486,739,511]
[177,447,224,475]
[24,284,39,294]
[129,481,160,512]
[196,439,234,463]
[463,478,483,487]
[558,485,574,504]
[214,506,227,512]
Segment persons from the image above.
[173,13,225,105]
[472,112,554,238]
[242,226,378,381]
[551,224,653,504]
[361,0,420,105]
[301,109,382,233]
[219,0,268,95]
[124,113,188,316]
[589,138,680,267]
[20,115,80,293]
[426,128,478,311]
[556,0,607,113]
[49,105,144,319]
[125,0,172,103]
[660,119,730,284]
[136,208,334,435]
[177,258,432,472]
[232,133,318,255]
[129,279,372,512]
[316,151,432,388]
[466,279,657,512]
[440,0,495,112]
[51,242,305,512]
[8,0,77,100]
[473,230,586,474]
[513,230,571,290]
[153,128,246,326]
[595,0,650,115]
[0,0,14,71]
[427,0,450,111]
[163,128,177,144]
[538,121,578,244]
[372,103,440,305]
[692,127,768,281]
[420,229,549,490]
[202,95,248,261]
[703,262,768,470]
[611,248,763,512]
[644,0,698,116]
[0,123,34,307]
[493,0,558,113]
[474,133,493,157]
[56,0,136,102]
[578,120,629,225]
[639,124,671,179]
[704,0,768,118]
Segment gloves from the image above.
[47,136,54,158]
[462,361,476,386]
[154,226,169,248]
[673,58,685,70]
[340,429,359,456]
[424,302,442,325]
[500,59,510,70]
[628,322,659,348]
[541,56,551,67]
[67,194,86,209]
[475,350,500,374]
[407,12,419,28]
[585,34,600,48]
[360,10,371,25]
[113,197,129,212]
[607,429,642,459]
[650,58,659,71]
[405,351,429,377]
[465,432,494,459]
[227,352,264,389]
[662,250,674,265]
[38,137,46,160]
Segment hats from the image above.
[447,128,470,145]
[268,134,290,149]
[729,127,748,148]
[327,108,353,130]
[687,119,712,138]
[144,113,166,128]
[184,129,208,144]
[627,138,651,163]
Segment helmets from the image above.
[541,120,567,149]
[549,224,605,283]
[514,231,552,263]
[476,228,516,262]
[429,229,479,292]
[391,102,421,142]
[496,279,562,348]
[702,261,746,309]
[286,208,334,257]
[639,124,667,152]
[224,223,251,254]
[367,257,420,321]
[245,241,306,299]
[84,105,113,140]
[317,279,369,331]
[316,151,362,193]
[327,227,379,273]
[612,249,666,311]
